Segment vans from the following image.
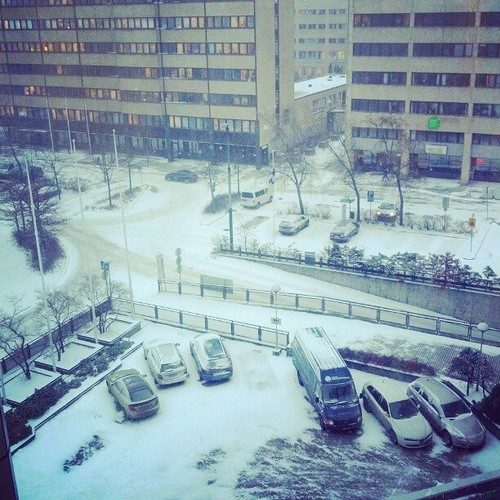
[239,186,274,210]
[0,156,28,179]
[376,197,401,223]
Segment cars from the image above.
[165,170,198,184]
[407,377,487,449]
[279,213,309,237]
[360,377,433,448]
[189,331,233,386]
[106,368,160,422]
[329,220,360,243]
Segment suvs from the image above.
[142,337,189,386]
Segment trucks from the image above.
[290,325,365,431]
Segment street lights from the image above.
[475,322,490,392]
[269,284,282,357]
